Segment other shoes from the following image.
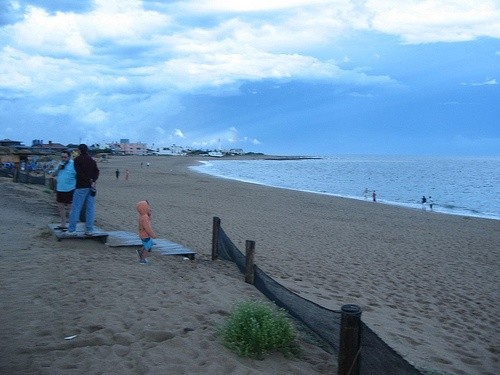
[54,227,68,231]
[139,260,148,265]
[61,231,77,237]
[77,222,86,226]
[56,225,62,228]
[136,248,143,258]
[84,231,93,235]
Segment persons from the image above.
[136,200,157,264]
[115,169,129,181]
[372,190,376,201]
[364,188,368,200]
[48,144,99,236]
[421,196,433,213]
